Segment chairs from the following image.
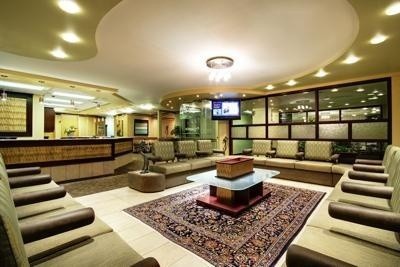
[0,152,158,267]
[143,138,224,188]
[244,139,337,187]
[285,142,400,267]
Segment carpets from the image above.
[124,183,327,267]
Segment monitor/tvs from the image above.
[211,98,241,120]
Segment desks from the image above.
[128,170,165,194]
